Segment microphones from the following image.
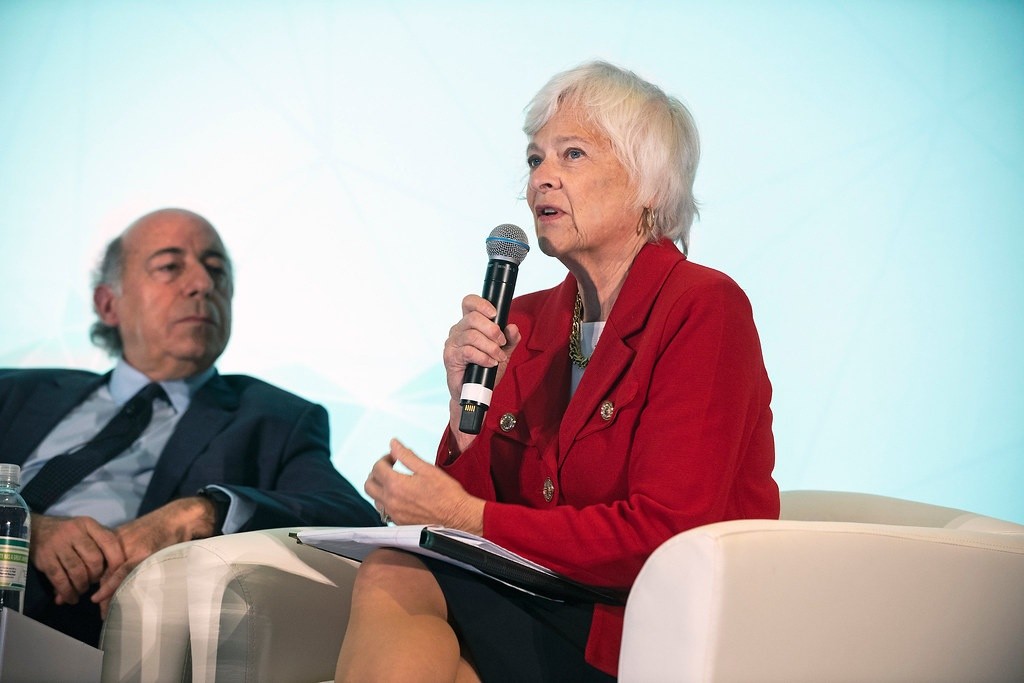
[456,224,530,436]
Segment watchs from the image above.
[193,487,231,536]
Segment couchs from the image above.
[97,491,1024,683]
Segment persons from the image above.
[0,208,389,650]
[334,60,781,683]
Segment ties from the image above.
[19,382,166,515]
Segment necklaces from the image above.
[570,291,590,370]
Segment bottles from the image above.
[0,463,32,618]
